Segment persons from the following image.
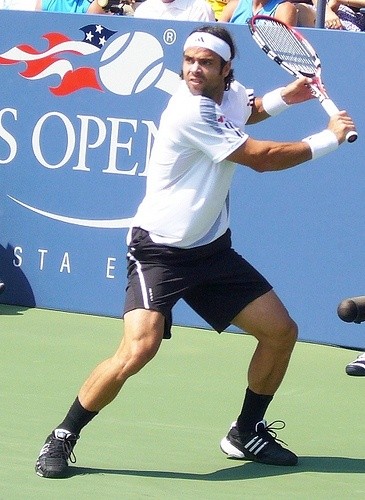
[34,27,358,480]
[0,0,365,33]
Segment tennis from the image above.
[99,31,164,96]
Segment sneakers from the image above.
[35,429,80,478]
[220,419,298,465]
[346,353,365,376]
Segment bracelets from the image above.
[262,87,293,117]
[301,129,340,160]
